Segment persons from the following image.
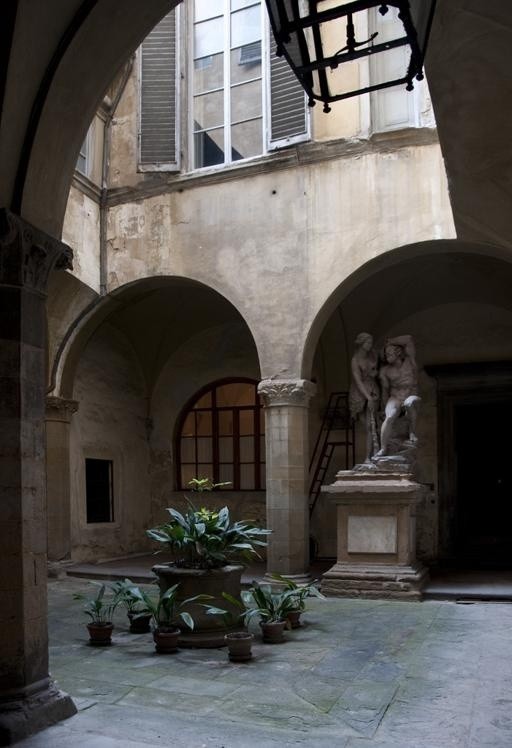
[371,334,422,461]
[351,332,380,465]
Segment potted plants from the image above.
[142,476,275,652]
[107,579,153,635]
[73,577,126,650]
[123,583,229,654]
[239,579,290,644]
[264,570,328,630]
[193,590,265,663]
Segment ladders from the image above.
[306,389,356,517]
